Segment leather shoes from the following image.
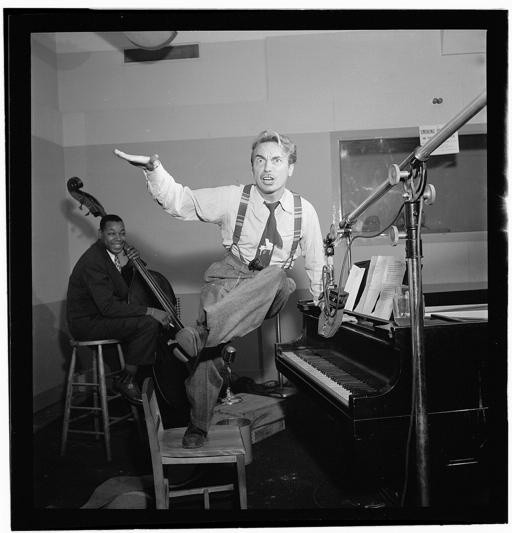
[167,330,200,364]
[181,422,206,449]
[113,371,144,405]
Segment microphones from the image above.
[222,345,237,364]
[317,287,349,338]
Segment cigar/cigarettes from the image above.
[168,322,176,328]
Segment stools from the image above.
[58,336,141,462]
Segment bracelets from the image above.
[150,306,156,317]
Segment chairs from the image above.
[142,378,248,510]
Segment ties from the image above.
[114,254,121,273]
[251,201,280,271]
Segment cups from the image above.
[392,285,425,328]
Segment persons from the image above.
[111,129,359,449]
[65,213,174,407]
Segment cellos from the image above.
[67,176,193,411]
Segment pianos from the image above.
[275,281,489,508]
